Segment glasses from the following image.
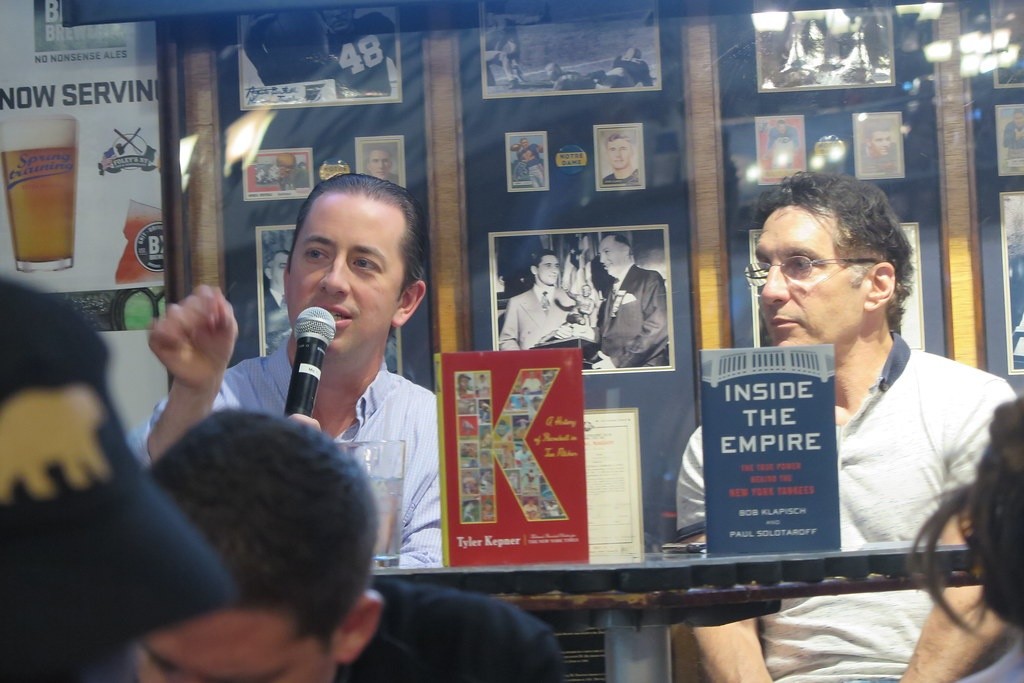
[742,255,877,287]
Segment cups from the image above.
[0,113,80,273]
[335,441,405,570]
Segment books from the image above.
[699,342,841,558]
[434,348,590,567]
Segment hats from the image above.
[0,277,228,659]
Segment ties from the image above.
[280,294,287,314]
[540,292,551,315]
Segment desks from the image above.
[389,567,980,683]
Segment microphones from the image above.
[282,306,336,422]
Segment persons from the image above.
[512,138,544,188]
[244,2,398,95]
[366,144,399,184]
[1003,110,1024,157]
[146,173,439,569]
[862,125,897,170]
[604,133,639,184]
[767,119,800,166]
[487,38,657,91]
[499,233,668,370]
[675,167,1017,682]
[1,279,568,682]
